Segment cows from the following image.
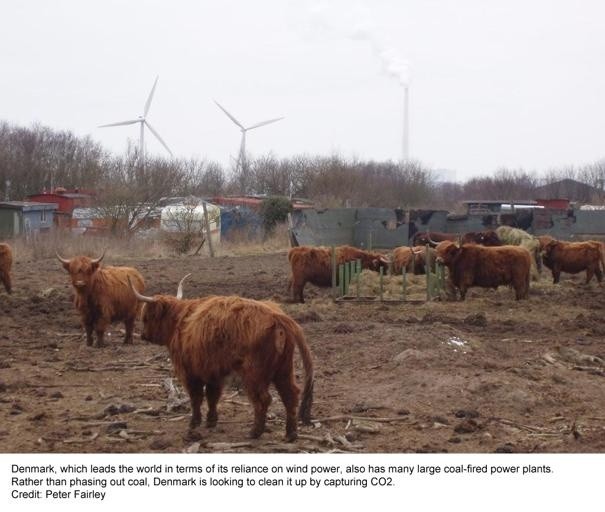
[393,225,541,301]
[55,248,145,348]
[287,245,394,303]
[532,235,605,286]
[127,272,315,442]
[0,242,14,294]
